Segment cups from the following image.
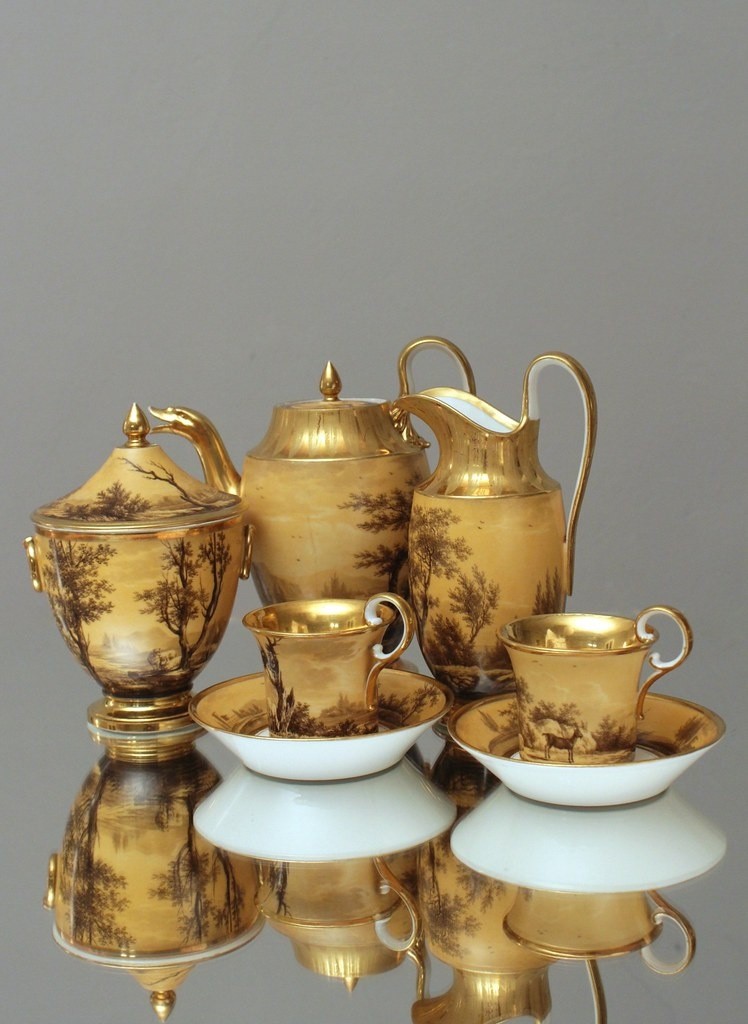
[494,603,694,764]
[501,882,695,979]
[239,592,419,734]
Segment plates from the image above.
[187,666,453,784]
[186,756,456,871]
[445,687,726,811]
[449,786,727,894]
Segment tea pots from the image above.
[147,330,476,674]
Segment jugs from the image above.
[411,744,617,1024]
[394,352,599,693]
